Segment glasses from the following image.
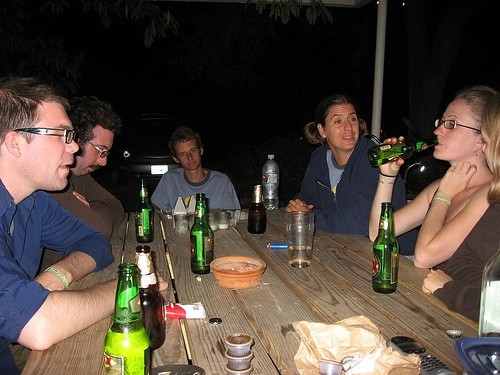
[14,127,76,144]
[434,119,482,132]
[87,140,109,159]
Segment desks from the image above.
[20,205,478,375]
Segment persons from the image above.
[149,130,241,212]
[369,85,500,321]
[0,78,168,375]
[286,94,419,261]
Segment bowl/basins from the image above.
[224,350,253,371]
[319,359,343,375]
[209,256,267,289]
[224,333,253,357]
[224,363,253,375]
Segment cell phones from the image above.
[386,336,457,375]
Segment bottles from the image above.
[103,263,150,375]
[262,155,279,212]
[372,202,399,294]
[134,245,166,351]
[478,248,500,337]
[248,184,267,235]
[135,176,155,243]
[367,138,439,169]
[190,193,214,275]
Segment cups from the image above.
[285,210,314,270]
[172,208,242,234]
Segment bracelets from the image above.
[378,172,396,184]
[432,196,451,205]
[44,267,69,288]
